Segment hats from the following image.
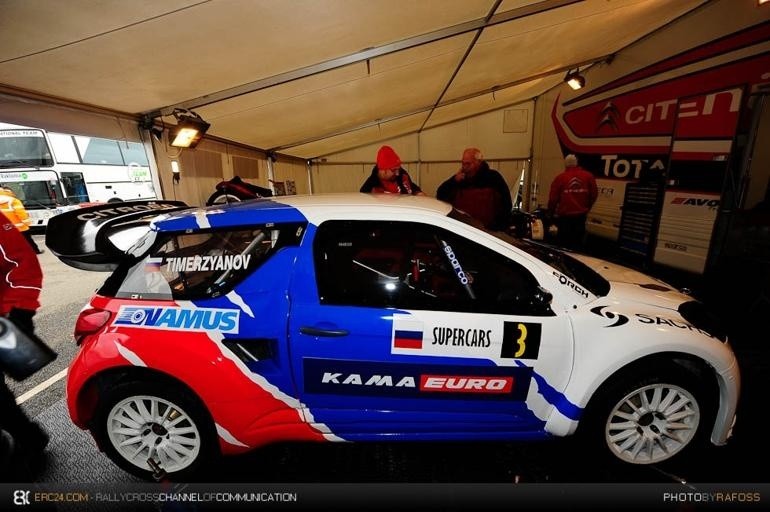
[564,153,579,169]
[375,144,402,170]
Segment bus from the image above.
[0,127,155,232]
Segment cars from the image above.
[66,189,740,486]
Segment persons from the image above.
[360,145,427,197]
[436,148,512,231]
[0,187,44,483]
[547,155,598,255]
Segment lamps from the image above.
[564,67,585,91]
[168,108,211,148]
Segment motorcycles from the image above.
[0,314,60,485]
